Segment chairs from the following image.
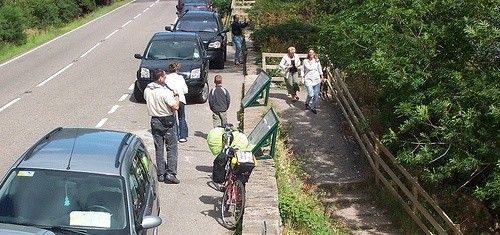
[85,178,122,219]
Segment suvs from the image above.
[0,127,163,235]
[165,9,230,69]
[175,0,213,17]
[134,31,214,103]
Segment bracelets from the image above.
[175,94,179,96]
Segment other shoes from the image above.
[292,97,297,101]
[179,138,186,142]
[158,174,165,182]
[295,95,298,99]
[235,60,239,65]
[311,109,317,114]
[306,104,308,108]
[165,174,180,184]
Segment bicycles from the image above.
[210,123,256,230]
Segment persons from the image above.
[164,63,189,142]
[143,69,180,184]
[231,15,250,65]
[208,75,230,128]
[301,48,323,114]
[279,47,301,101]
